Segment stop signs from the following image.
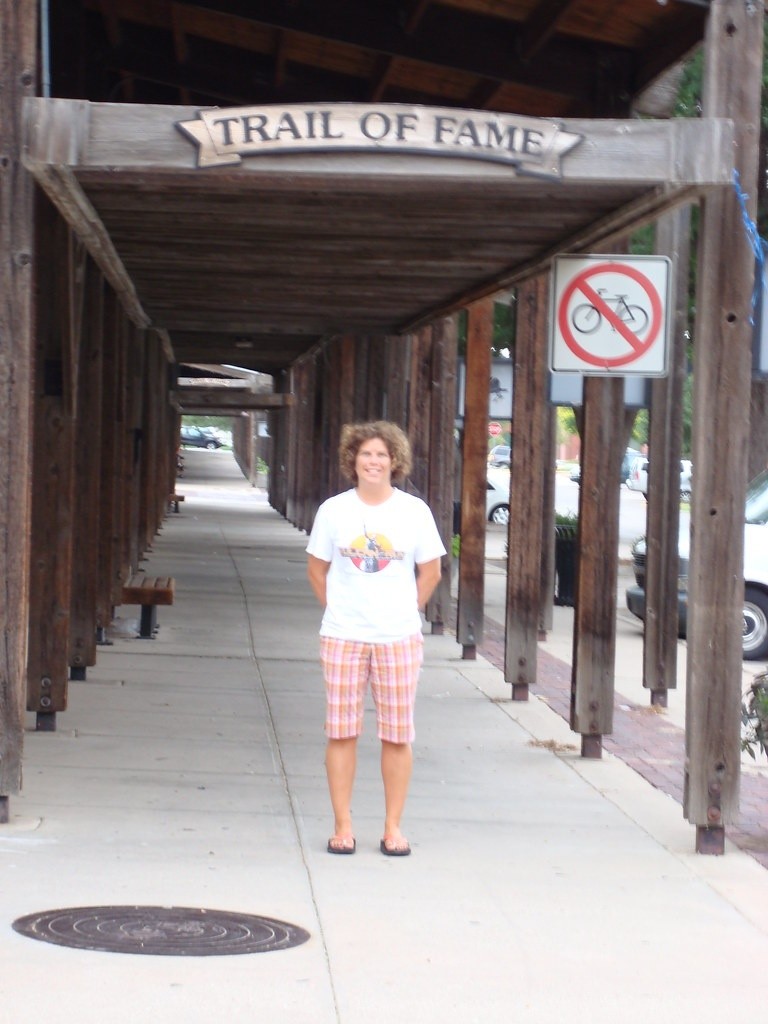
[489,423,501,436]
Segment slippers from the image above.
[327,834,356,852]
[380,837,411,854]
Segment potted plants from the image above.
[555,512,578,607]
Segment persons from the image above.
[305,421,448,856]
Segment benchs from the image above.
[121,574,175,639]
[169,494,184,513]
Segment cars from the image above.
[180,426,223,450]
[487,446,511,468]
[627,468,768,663]
[569,453,648,485]
[626,459,693,503]
[486,476,510,525]
